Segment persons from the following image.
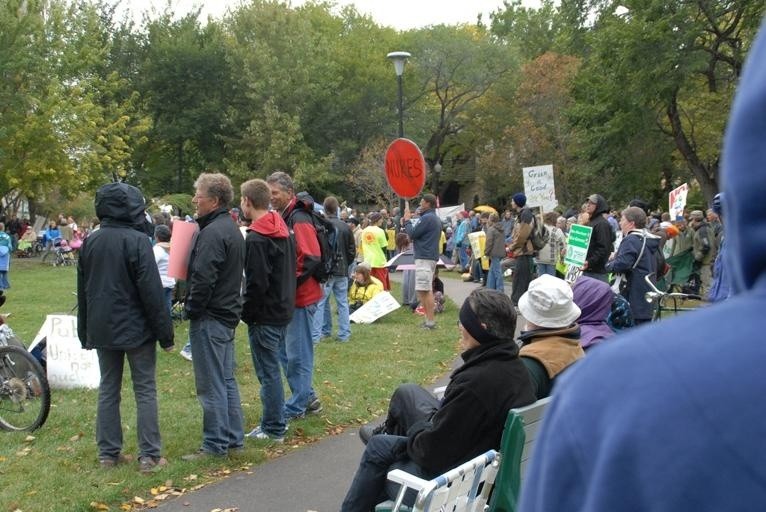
[339,288,537,512]
[523,20,766,511]
[77,173,328,474]
[358,272,587,445]
[1,193,724,345]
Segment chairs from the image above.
[372,448,502,512]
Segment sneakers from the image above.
[180,350,193,361]
[305,398,323,415]
[360,424,386,445]
[139,456,168,478]
[285,412,306,424]
[417,322,439,330]
[181,449,228,462]
[244,426,285,444]
[227,447,245,456]
[99,454,133,467]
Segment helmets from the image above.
[711,192,730,216]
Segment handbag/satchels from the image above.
[609,271,632,297]
[69,237,81,248]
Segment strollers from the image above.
[48,238,79,268]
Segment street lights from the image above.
[386,51,413,219]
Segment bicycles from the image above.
[643,271,703,323]
[0,345,50,434]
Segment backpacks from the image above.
[288,208,340,283]
[530,215,550,250]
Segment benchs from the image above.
[487,397,551,512]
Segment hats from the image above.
[367,213,382,222]
[518,274,582,328]
[676,216,684,222]
[460,211,469,218]
[688,210,704,220]
[585,194,598,204]
[60,240,67,245]
[512,193,526,208]
